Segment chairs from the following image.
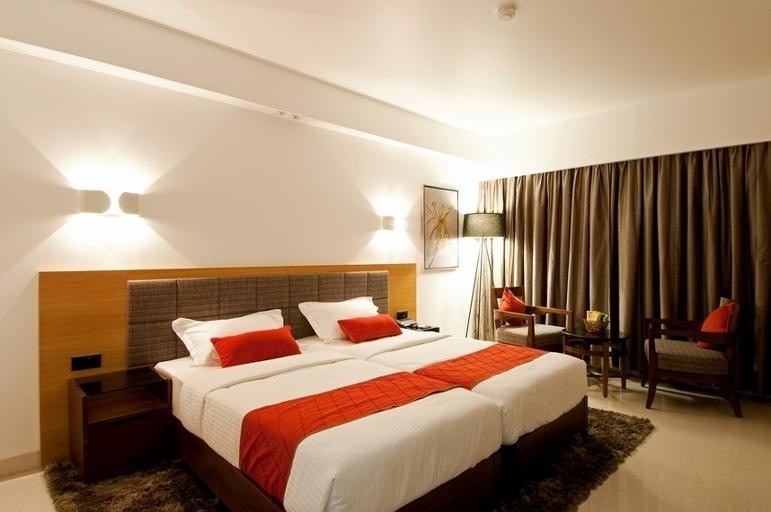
[490,286,573,348]
[641,297,745,417]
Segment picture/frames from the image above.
[422,184,460,269]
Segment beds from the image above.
[126,270,590,512]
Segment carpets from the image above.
[45,396,654,511]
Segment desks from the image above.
[561,329,629,398]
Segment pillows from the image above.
[337,313,401,343]
[210,325,302,369]
[696,301,739,348]
[298,296,378,341]
[500,288,525,327]
[172,308,285,367]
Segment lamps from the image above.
[462,213,504,339]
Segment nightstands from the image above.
[69,365,173,465]
[397,319,439,334]
